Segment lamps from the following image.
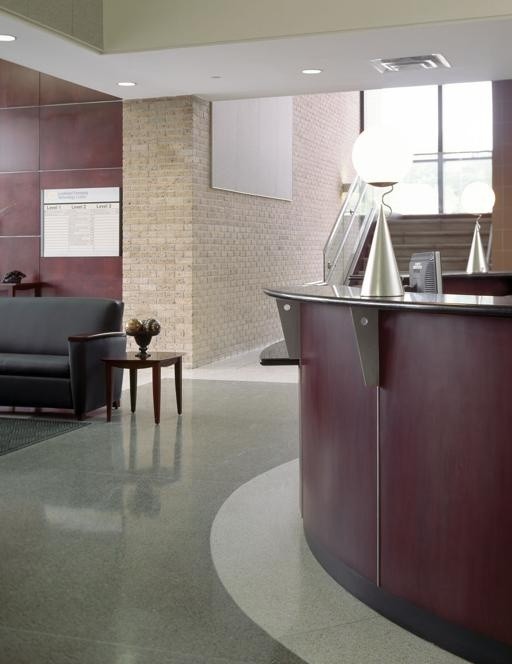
[352,123,412,298]
[462,181,498,274]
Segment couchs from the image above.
[0,298,125,419]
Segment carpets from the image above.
[1,413,91,463]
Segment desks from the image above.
[0,281,44,297]
[231,269,512,660]
[100,351,186,423]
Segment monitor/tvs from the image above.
[402,250,444,295]
